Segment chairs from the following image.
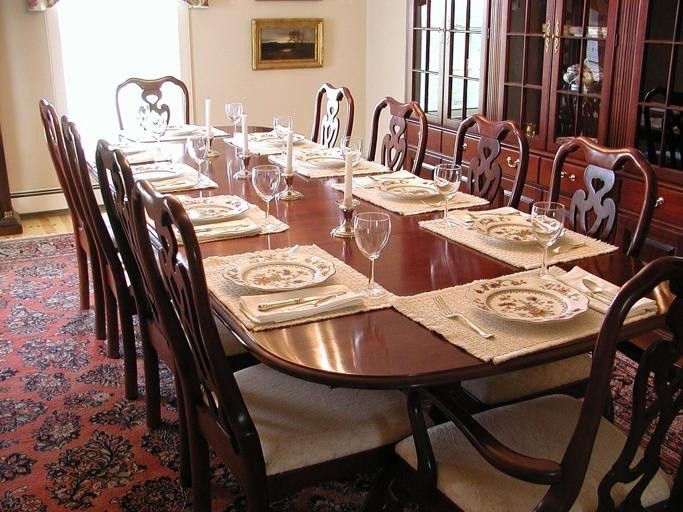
[391,253,683,510]
[37,98,109,341]
[126,177,422,510]
[441,108,531,213]
[57,114,144,402]
[94,140,197,491]
[307,81,357,158]
[111,77,194,131]
[543,133,663,260]
[363,95,432,179]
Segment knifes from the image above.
[255,291,347,311]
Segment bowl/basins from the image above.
[540,21,608,38]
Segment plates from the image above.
[180,195,248,225]
[247,131,359,168]
[117,145,183,179]
[464,275,591,324]
[378,177,441,201]
[471,214,541,247]
[223,253,335,291]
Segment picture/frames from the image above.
[251,18,325,71]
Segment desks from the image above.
[69,106,680,395]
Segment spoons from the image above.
[580,276,621,300]
[367,174,415,182]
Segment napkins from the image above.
[537,264,658,323]
[168,215,268,245]
[232,282,369,324]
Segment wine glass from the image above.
[224,103,243,136]
[186,135,210,187]
[529,199,566,278]
[352,210,393,301]
[252,165,282,228]
[433,164,463,229]
[148,115,166,147]
[271,114,296,148]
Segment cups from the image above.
[341,137,363,167]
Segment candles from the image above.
[330,149,362,242]
[275,112,305,202]
[199,96,222,157]
[231,110,256,181]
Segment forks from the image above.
[545,240,586,257]
[430,293,493,340]
